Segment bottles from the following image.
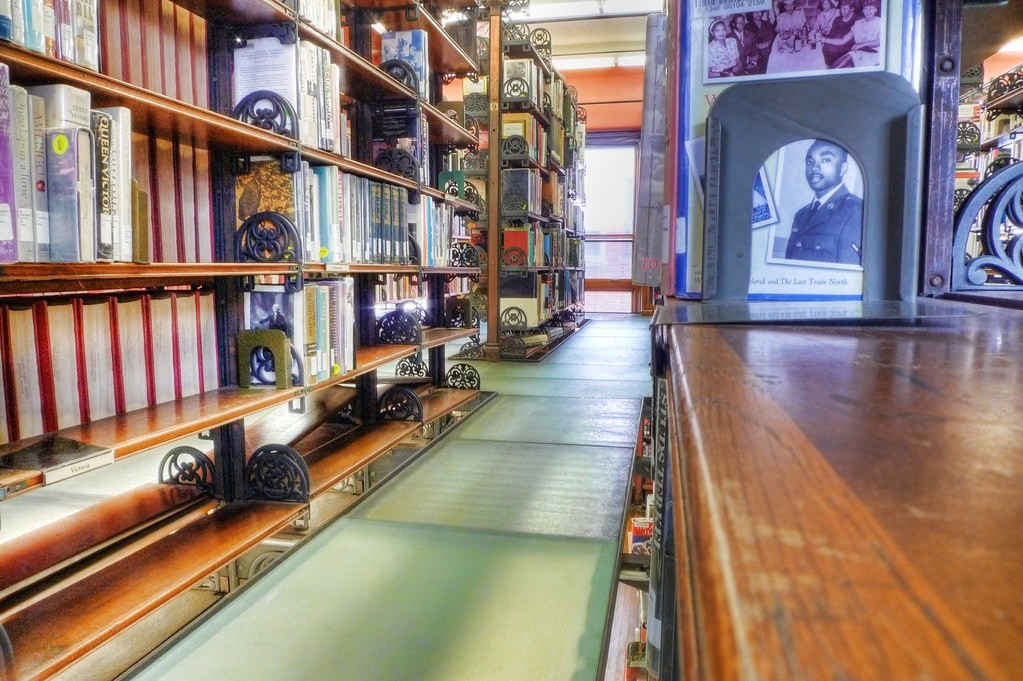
[778,39,785,53]
[802,22,808,39]
[794,33,802,52]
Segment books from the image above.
[955,99,1023,285]
[624,379,677,681]
[0,0,472,498]
[463,58,582,347]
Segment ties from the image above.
[808,201,821,222]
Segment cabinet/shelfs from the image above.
[978,85,1023,151]
[0,0,499,681]
[956,143,981,180]
[448,0,592,364]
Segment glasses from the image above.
[736,22,745,26]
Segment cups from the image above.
[811,34,817,49]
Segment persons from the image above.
[255,304,286,371]
[708,0,881,78]
[785,140,863,265]
[238,166,268,260]
